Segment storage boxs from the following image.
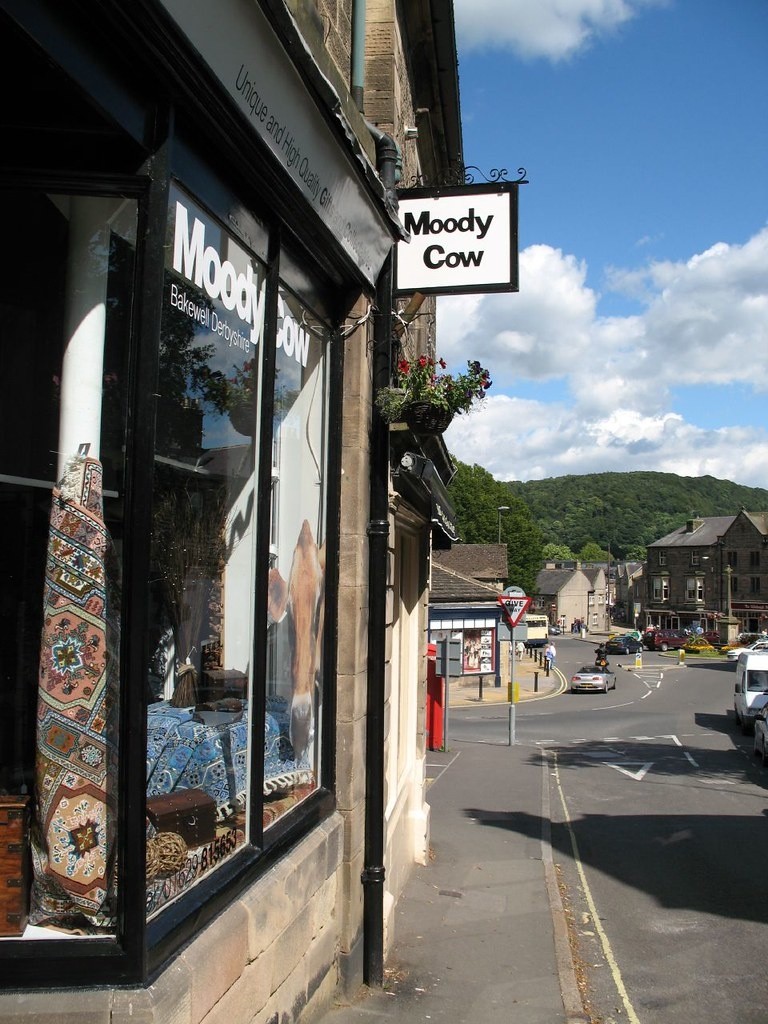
[204,670,248,702]
[147,788,217,847]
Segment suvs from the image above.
[643,629,691,652]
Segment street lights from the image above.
[587,591,595,627]
[498,506,510,546]
[699,556,709,570]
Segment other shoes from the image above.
[551,668,554,670]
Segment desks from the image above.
[147,695,314,823]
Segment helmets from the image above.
[600,643,604,647]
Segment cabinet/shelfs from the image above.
[0,795,34,938]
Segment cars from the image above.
[698,631,721,642]
[549,624,561,635]
[726,641,768,662]
[570,666,616,693]
[606,636,644,654]
[739,633,766,643]
[753,701,768,763]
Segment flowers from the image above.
[374,354,493,425]
[683,634,715,651]
[220,358,281,414]
[721,640,748,651]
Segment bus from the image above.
[520,614,548,648]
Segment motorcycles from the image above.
[594,650,609,669]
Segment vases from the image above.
[407,403,455,434]
[227,404,256,436]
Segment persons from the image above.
[545,642,556,670]
[516,642,525,661]
[595,643,608,661]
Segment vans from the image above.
[733,651,768,732]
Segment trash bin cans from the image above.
[581,628,585,638]
